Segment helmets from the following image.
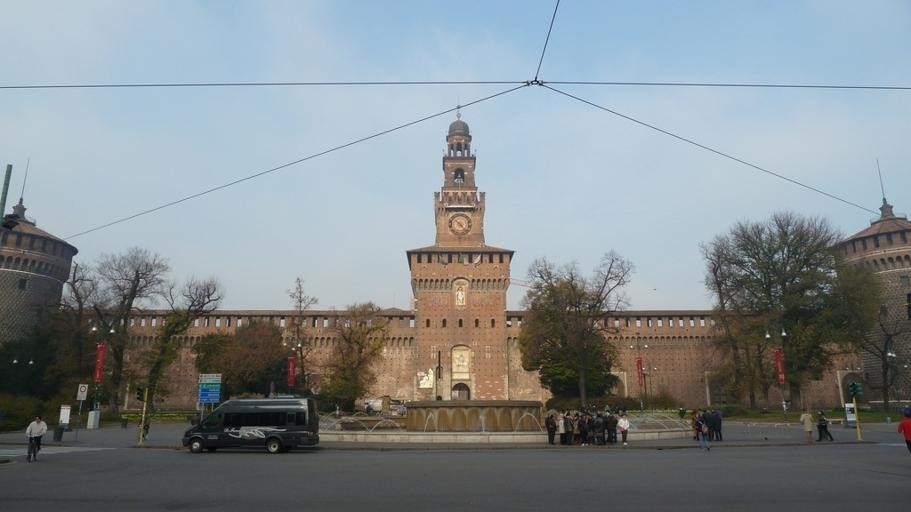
[706,439,722,441]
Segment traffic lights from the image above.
[136,387,145,401]
[847,382,863,399]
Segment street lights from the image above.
[629,335,658,410]
[91,318,117,410]
[765,321,791,424]
[283,342,303,396]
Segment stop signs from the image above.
[77,383,89,402]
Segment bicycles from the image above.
[26,434,41,463]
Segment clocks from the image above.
[448,212,473,237]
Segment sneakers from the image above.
[830,439,833,441]
[816,440,821,442]
[701,447,711,451]
[548,442,628,446]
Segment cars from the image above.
[366,396,402,412]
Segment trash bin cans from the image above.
[121,418,128,429]
[679,408,686,418]
[191,416,201,425]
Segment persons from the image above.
[815,409,833,442]
[456,286,465,305]
[545,400,630,448]
[363,401,372,418]
[897,408,911,453]
[799,407,814,444]
[679,405,686,419]
[23,415,47,461]
[399,402,405,416]
[137,410,150,441]
[690,407,722,452]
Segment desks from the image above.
[827,419,844,425]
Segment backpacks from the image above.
[699,421,708,434]
[698,438,700,441]
[693,436,697,440]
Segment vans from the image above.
[181,395,319,454]
